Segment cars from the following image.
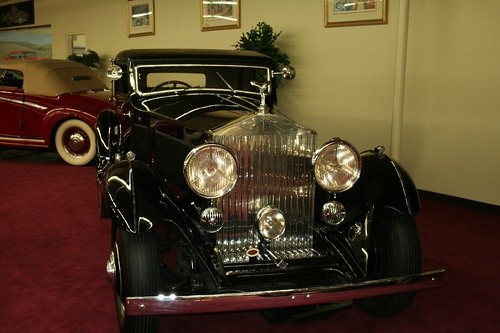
[0,58,132,166]
[94,48,447,333]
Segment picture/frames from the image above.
[199,0,241,32]
[126,0,155,38]
[323,0,389,28]
